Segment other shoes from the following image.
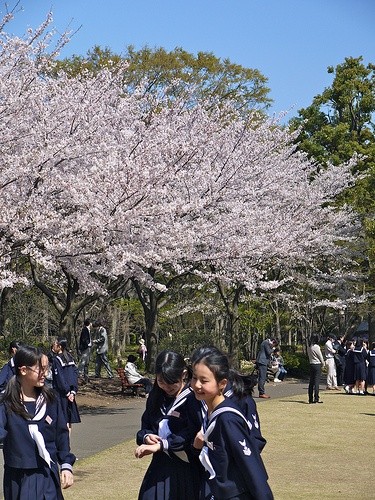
[327,386,335,390]
[309,400,314,403]
[314,400,323,403]
[333,387,339,390]
[259,393,271,398]
[274,378,281,382]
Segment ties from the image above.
[199,417,217,479]
[18,391,56,468]
[158,387,193,439]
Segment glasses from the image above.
[18,366,44,378]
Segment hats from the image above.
[127,355,137,363]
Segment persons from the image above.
[325,333,375,396]
[124,354,153,398]
[190,349,276,500]
[91,320,114,379]
[0,340,28,394]
[255,337,281,398]
[268,347,287,382]
[79,318,93,379]
[0,346,76,500]
[136,338,147,363]
[191,344,262,449]
[134,350,213,500]
[51,337,81,438]
[307,332,325,404]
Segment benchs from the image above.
[118,368,144,397]
